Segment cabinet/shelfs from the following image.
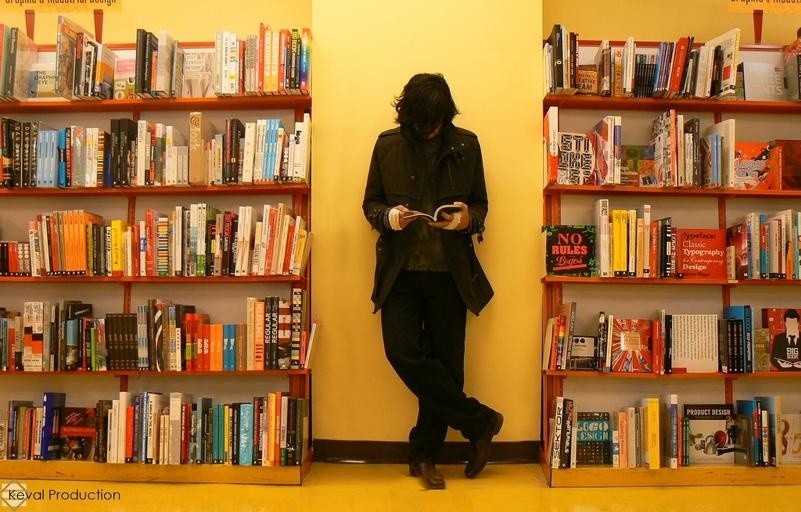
[1,28,314,484]
[541,26,800,488]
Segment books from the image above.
[399,202,462,224]
[544,23,801,471]
[0,15,312,468]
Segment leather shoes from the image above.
[464,407,503,478]
[408,452,445,489]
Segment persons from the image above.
[362,73,504,489]
